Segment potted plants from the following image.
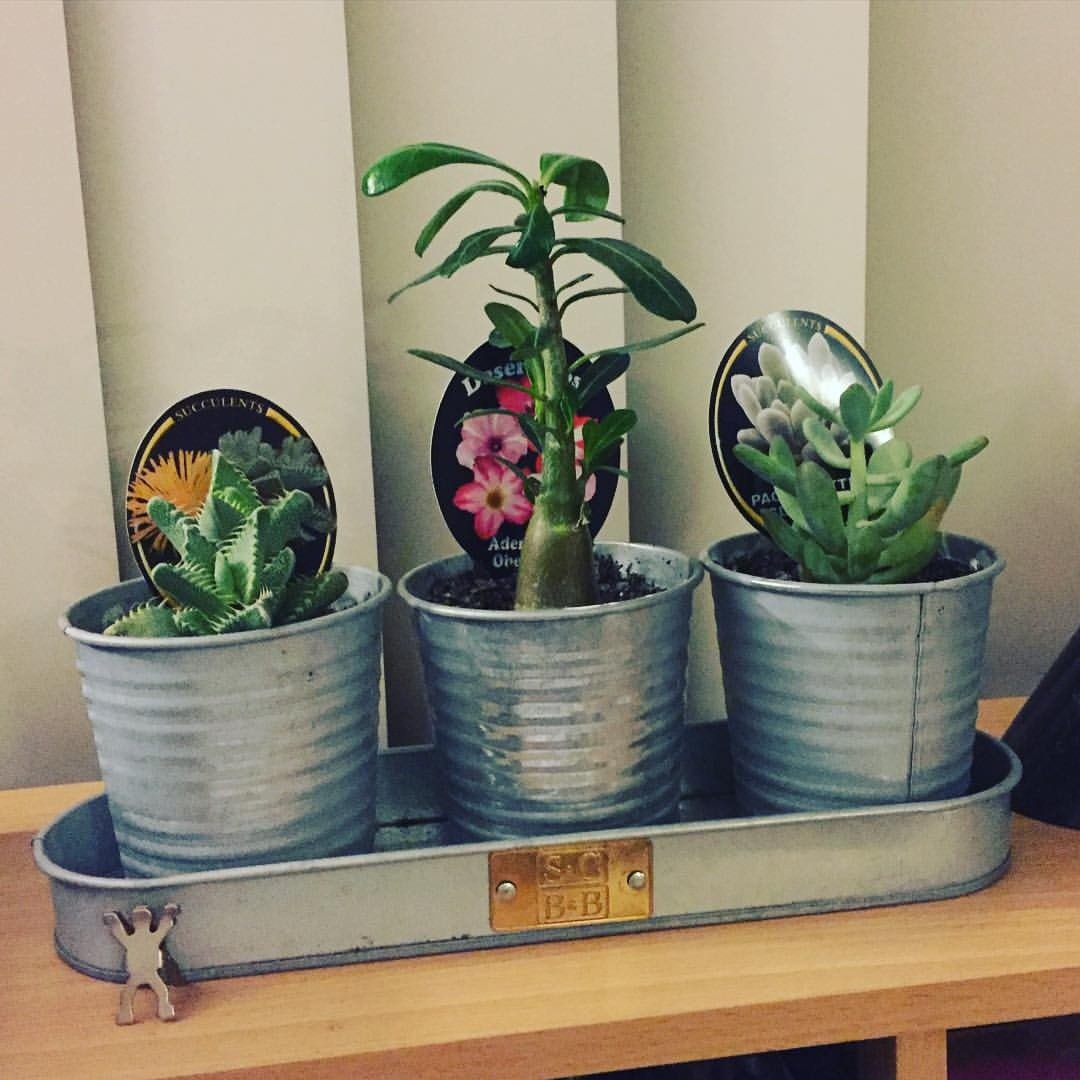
[60,424,393,880]
[700,330,1000,809]
[362,132,705,845]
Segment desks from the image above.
[0,698,1080,1080]
